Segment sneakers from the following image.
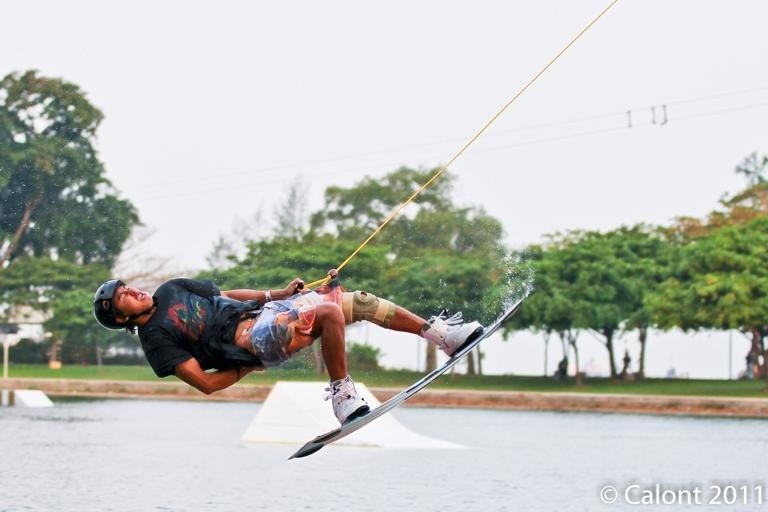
[330,375,369,425]
[419,315,483,357]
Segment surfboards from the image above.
[292,280,535,459]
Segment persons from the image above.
[92,277,485,426]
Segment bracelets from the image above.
[235,367,240,382]
[264,290,272,302]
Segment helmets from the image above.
[95,280,125,329]
